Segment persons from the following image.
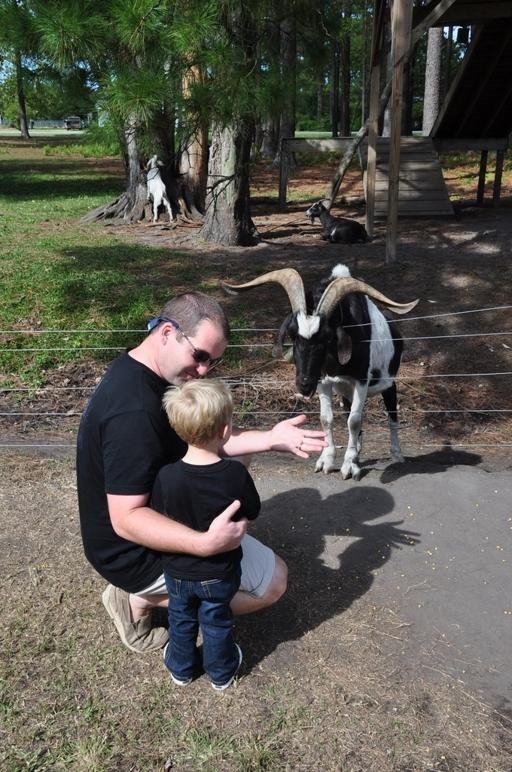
[77,291,329,653]
[149,378,261,690]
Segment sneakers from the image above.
[163,642,243,691]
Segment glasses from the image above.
[182,331,223,367]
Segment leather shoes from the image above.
[102,583,169,654]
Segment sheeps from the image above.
[220,262,423,481]
[305,198,373,244]
[146,154,174,224]
